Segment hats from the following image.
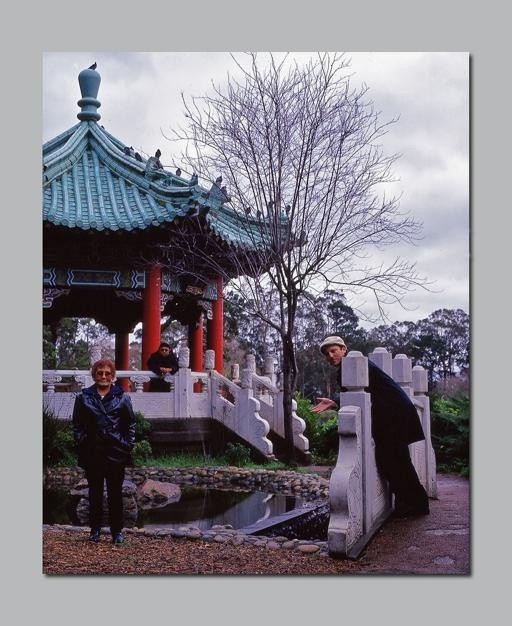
[319,336,347,356]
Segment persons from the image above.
[311,335,430,519]
[73,359,136,543]
[148,343,179,392]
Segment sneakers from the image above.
[112,532,124,546]
[88,531,100,542]
